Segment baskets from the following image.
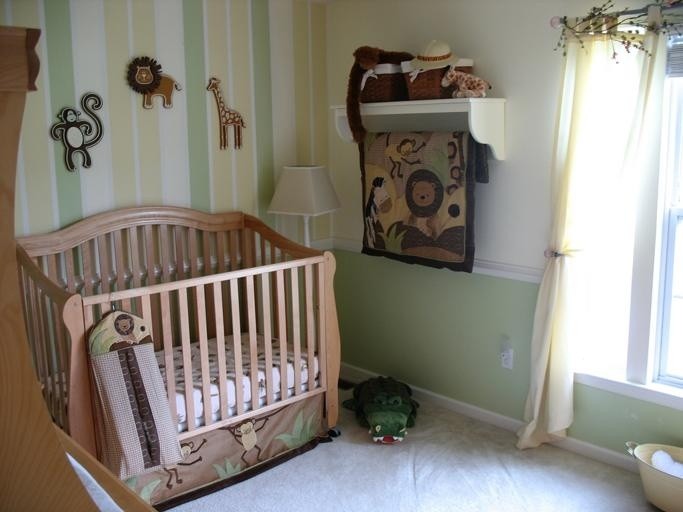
[359,57,474,103]
[624,441,683,512]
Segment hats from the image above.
[410,38,457,69]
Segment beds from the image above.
[13,205,343,505]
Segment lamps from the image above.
[266,163,341,250]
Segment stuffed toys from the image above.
[342,376,419,442]
[441,64,492,98]
